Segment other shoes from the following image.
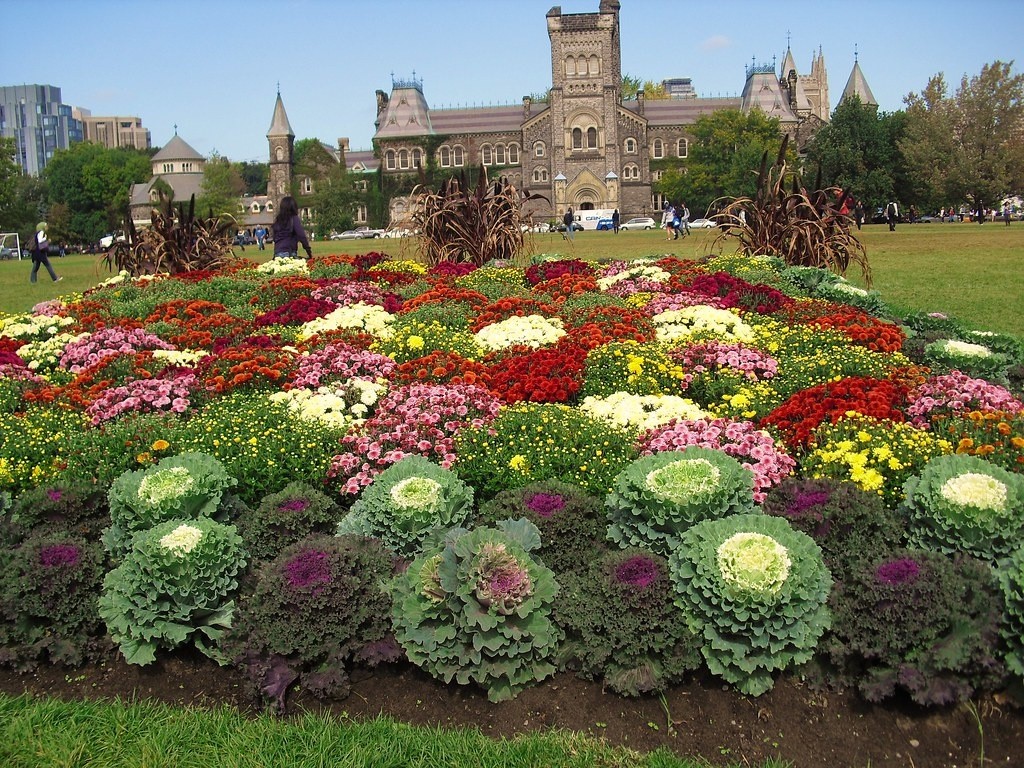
[666,232,686,241]
[53,276,63,282]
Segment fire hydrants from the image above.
[324,235,327,240]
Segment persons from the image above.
[716,202,747,234]
[537,220,542,233]
[673,203,686,240]
[271,196,313,262]
[238,223,270,251]
[563,207,574,241]
[840,197,1024,232]
[311,227,338,242]
[612,209,619,234]
[660,200,679,241]
[680,201,690,236]
[59,238,97,258]
[30,221,63,286]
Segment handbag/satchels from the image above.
[38,241,48,253]
[673,216,680,227]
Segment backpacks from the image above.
[888,203,896,217]
[676,204,685,218]
[25,234,37,253]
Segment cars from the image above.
[921,211,958,223]
[0,243,104,259]
[619,218,656,231]
[521,223,550,233]
[332,230,363,240]
[550,222,584,231]
[381,228,414,239]
[233,236,257,245]
[596,219,614,231]
[687,218,718,228]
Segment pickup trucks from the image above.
[354,227,384,238]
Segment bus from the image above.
[573,209,620,230]
